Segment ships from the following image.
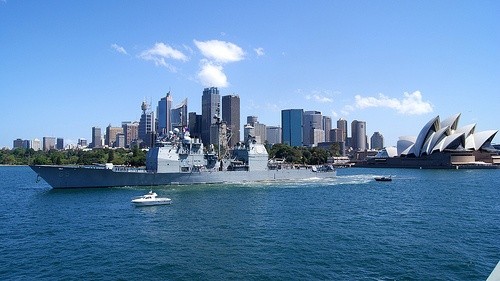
[28,126,338,190]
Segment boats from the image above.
[130,191,172,206]
[374,177,392,181]
